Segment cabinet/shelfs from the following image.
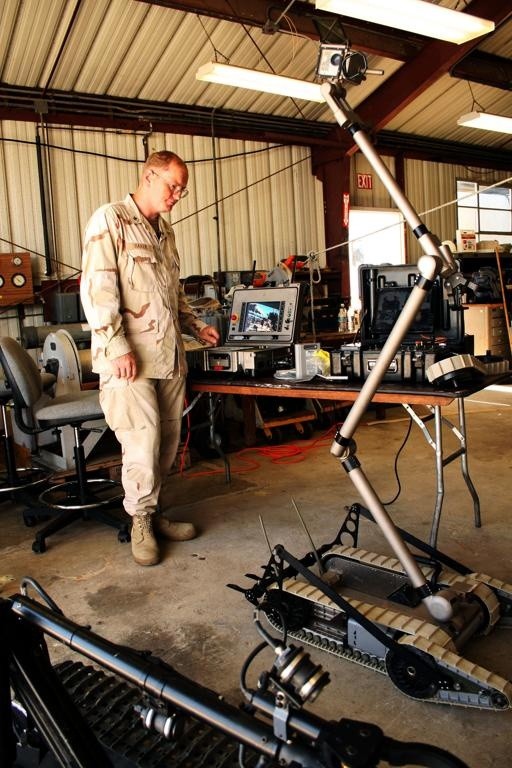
[461,303,510,357]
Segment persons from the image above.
[77,149,223,566]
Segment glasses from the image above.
[154,171,189,199]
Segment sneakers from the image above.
[130,515,196,566]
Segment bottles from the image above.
[337,303,355,333]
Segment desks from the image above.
[187,372,512,561]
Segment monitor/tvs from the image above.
[225,282,302,344]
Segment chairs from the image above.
[0,337,131,553]
[0,373,75,508]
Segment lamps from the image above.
[457,81,512,134]
[195,14,326,103]
[309,0,495,45]
